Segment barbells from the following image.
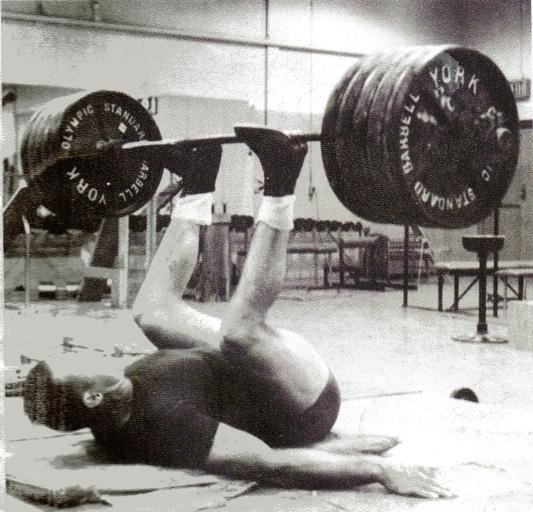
[23,44,519,227]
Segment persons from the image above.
[23,128,457,502]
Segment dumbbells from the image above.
[348,223,362,231]
[308,218,331,232]
[126,214,170,233]
[231,215,253,230]
[294,218,315,231]
[330,221,350,230]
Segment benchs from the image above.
[494,266,533,311]
[430,259,533,312]
